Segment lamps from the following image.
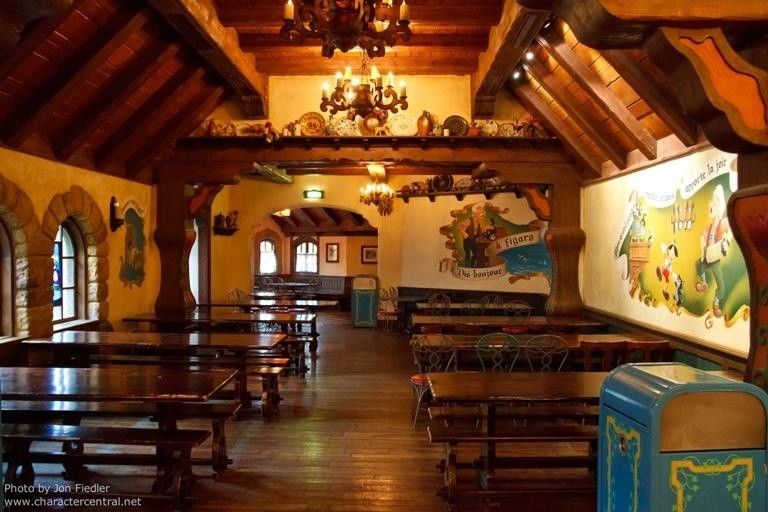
[278,0,413,116]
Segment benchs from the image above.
[421,423,600,509]
[1,328,323,512]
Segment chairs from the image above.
[227,274,322,331]
[374,286,672,429]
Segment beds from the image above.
[121,300,339,335]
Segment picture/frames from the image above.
[326,242,339,262]
[361,245,378,264]
